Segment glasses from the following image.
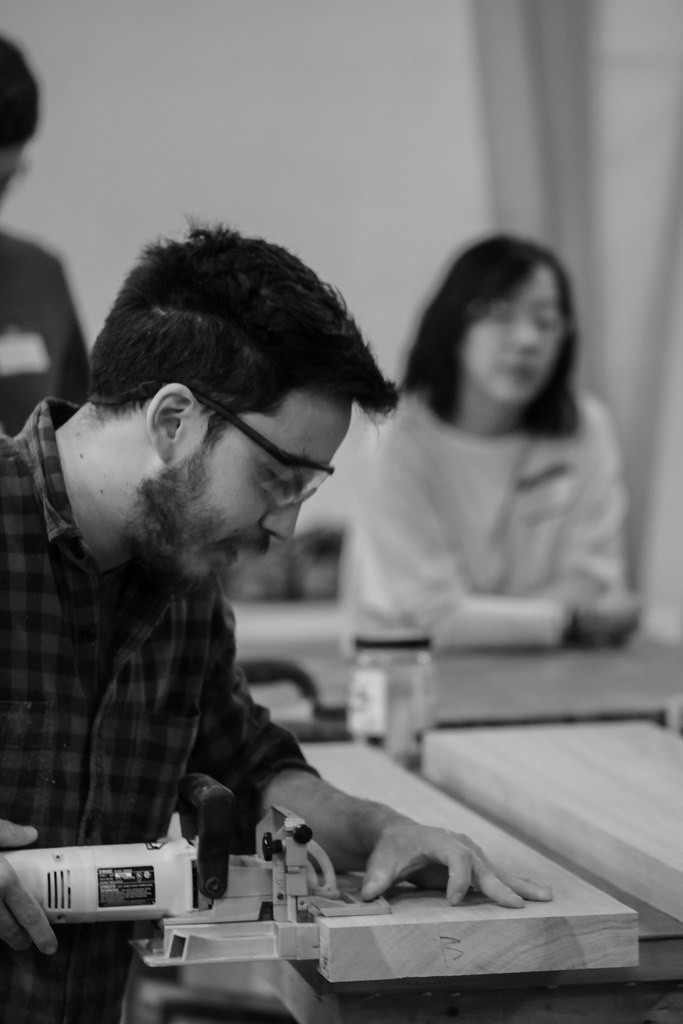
[194,396,335,506]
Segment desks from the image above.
[245,631,683,732]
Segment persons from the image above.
[0,37,91,440]
[334,236,641,647]
[0,220,553,1024]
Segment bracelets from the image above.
[559,601,590,648]
